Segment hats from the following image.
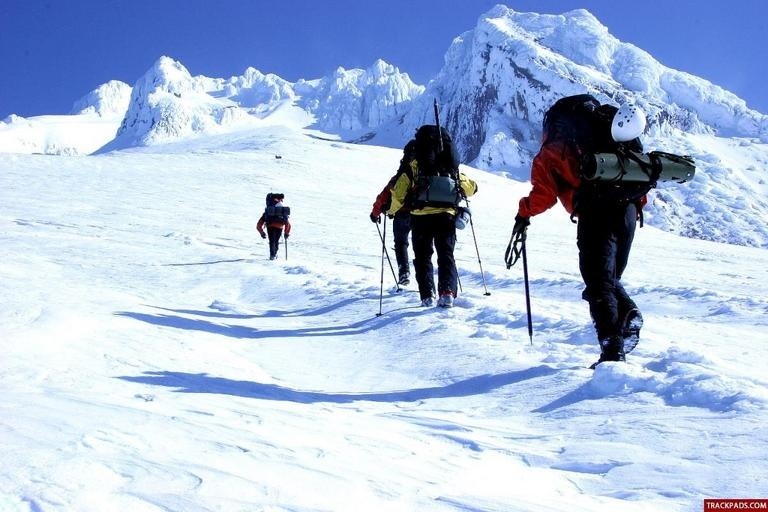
[403,139,416,153]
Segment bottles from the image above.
[455,207,471,230]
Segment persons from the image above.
[369,140,436,300]
[511,113,648,369]
[380,142,479,307]
[257,199,291,261]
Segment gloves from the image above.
[370,212,379,223]
[261,233,266,239]
[512,212,530,235]
[283,234,289,239]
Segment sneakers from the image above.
[420,290,454,308]
[270,255,278,260]
[397,272,410,286]
[589,304,644,370]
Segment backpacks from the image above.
[405,125,462,210]
[542,93,658,214]
[264,193,290,224]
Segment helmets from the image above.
[610,100,647,143]
